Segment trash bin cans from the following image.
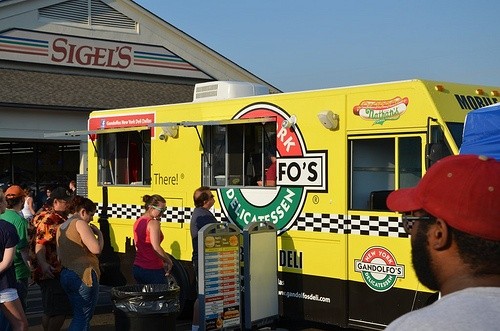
[112,284,181,331]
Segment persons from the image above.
[0,179,220,331]
[381,155,500,331]
[257,149,276,187]
[124,140,142,184]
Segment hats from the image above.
[5,185,27,199]
[50,186,70,201]
[385,154,499,239]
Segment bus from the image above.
[88,79,500,331]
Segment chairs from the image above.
[370,190,396,212]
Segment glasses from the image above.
[151,205,168,212]
[398,216,427,233]
